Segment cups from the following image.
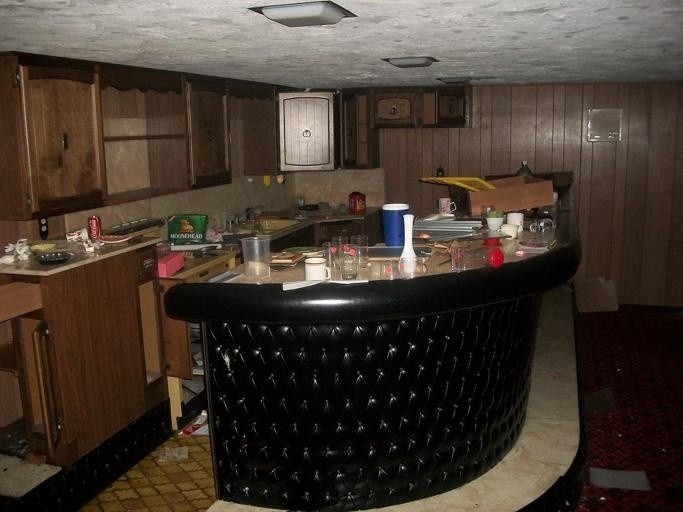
[286,191,367,218]
[438,198,456,215]
[65,227,89,243]
[481,204,524,239]
[529,218,556,238]
[304,235,506,281]
[240,236,270,278]
[382,203,409,246]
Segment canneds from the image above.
[87,214,102,242]
[65,227,89,243]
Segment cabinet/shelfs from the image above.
[186,73,233,191]
[3,50,106,219]
[228,78,381,178]
[0,203,382,467]
[100,62,191,203]
[372,83,473,130]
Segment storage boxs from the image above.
[466,175,553,218]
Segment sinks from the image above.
[243,219,300,236]
[350,247,432,257]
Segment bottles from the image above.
[397,214,417,274]
[436,166,443,179]
[219,212,269,235]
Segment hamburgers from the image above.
[178,224,194,234]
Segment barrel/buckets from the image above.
[382,202,410,246]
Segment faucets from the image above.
[246,205,264,220]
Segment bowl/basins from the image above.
[31,242,69,266]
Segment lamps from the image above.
[380,56,440,68]
[247,1,358,28]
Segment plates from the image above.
[99,234,131,244]
[414,217,483,233]
